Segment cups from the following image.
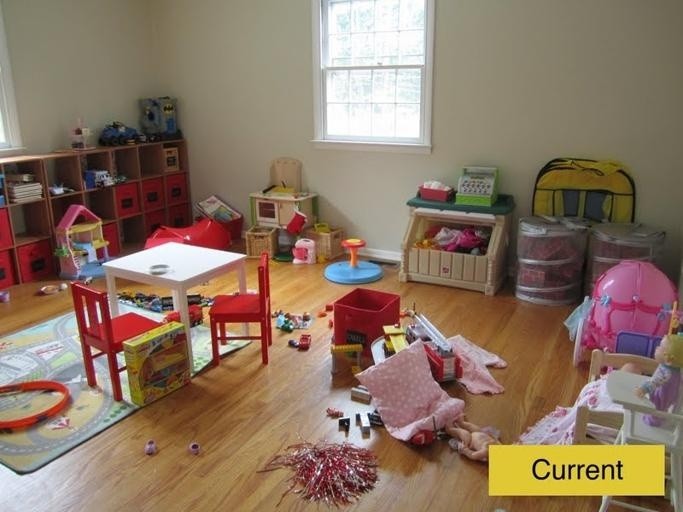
[0,291,8,302]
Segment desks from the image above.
[100,240,247,375]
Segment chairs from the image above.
[598,369,681,511]
[568,342,665,446]
[68,279,165,402]
[206,251,273,364]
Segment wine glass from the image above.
[81,128,89,150]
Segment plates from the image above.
[40,285,59,294]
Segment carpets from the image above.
[1,292,251,476]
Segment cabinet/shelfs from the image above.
[398,204,512,299]
[41,138,194,244]
[0,149,56,286]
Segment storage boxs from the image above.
[194,216,243,241]
[165,174,188,204]
[95,222,119,260]
[16,238,55,283]
[144,210,165,238]
[0,250,18,289]
[141,177,163,210]
[114,182,139,217]
[0,209,14,249]
[168,205,189,228]
[333,287,400,369]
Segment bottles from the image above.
[71,128,83,150]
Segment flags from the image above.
[634,334,682,426]
[563,259,682,372]
[0,167,505,464]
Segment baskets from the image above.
[307,225,345,260]
[246,226,277,259]
